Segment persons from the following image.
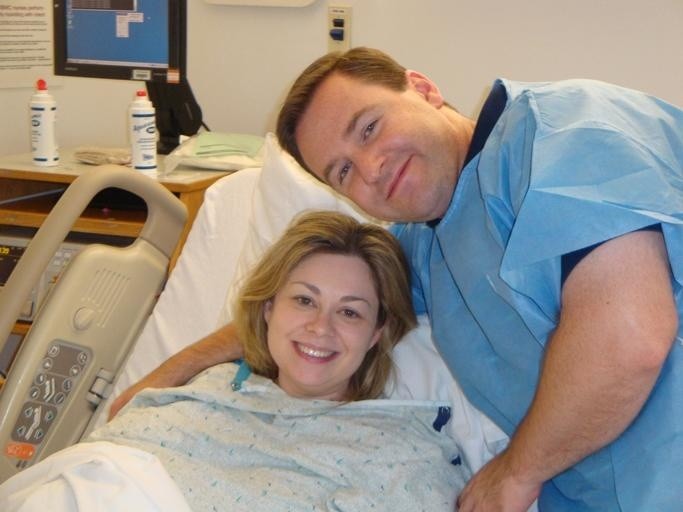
[106,47,683,512]
[79,211,471,512]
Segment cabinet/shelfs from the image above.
[0,147,234,387]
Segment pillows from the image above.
[216,129,508,470]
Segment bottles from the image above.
[30,79,59,166]
[129,89,157,179]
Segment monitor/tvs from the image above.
[53,0,188,87]
